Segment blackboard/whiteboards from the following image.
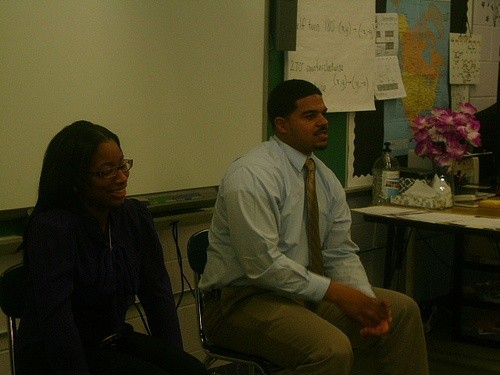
[0,0,268,220]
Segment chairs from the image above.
[0,263,27,375]
[187,230,272,375]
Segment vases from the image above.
[430,154,454,208]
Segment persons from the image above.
[198,79,429,375]
[13,120,211,375]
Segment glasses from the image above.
[86,159,134,179]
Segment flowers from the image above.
[411,102,481,165]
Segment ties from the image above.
[304,157,323,277]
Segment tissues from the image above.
[391,174,454,209]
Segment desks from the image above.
[348,197,500,350]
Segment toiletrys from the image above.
[371,141,401,207]
